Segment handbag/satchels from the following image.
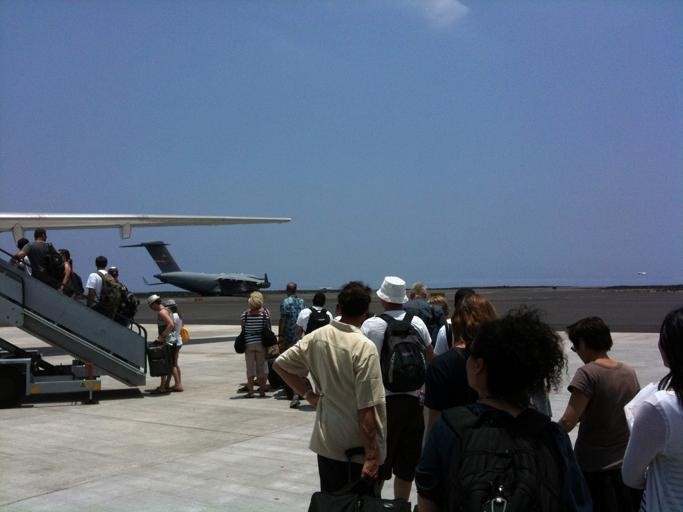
[261,306,277,347]
[232,310,247,354]
[180,329,189,343]
[61,272,83,297]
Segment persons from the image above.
[621,307,683,512]
[236,276,641,512]
[163,299,184,390]
[147,294,183,394]
[9,226,139,328]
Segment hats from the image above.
[164,299,177,308]
[146,294,160,305]
[375,274,410,305]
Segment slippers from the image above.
[150,386,184,395]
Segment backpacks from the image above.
[118,283,138,319]
[409,301,438,345]
[95,271,120,317]
[305,306,329,335]
[442,406,564,512]
[373,310,426,393]
[45,241,63,289]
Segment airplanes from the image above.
[119,240,271,298]
[0,210,295,243]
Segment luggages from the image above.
[306,446,412,512]
[147,340,175,377]
[264,351,286,389]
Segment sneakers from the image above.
[237,379,312,408]
